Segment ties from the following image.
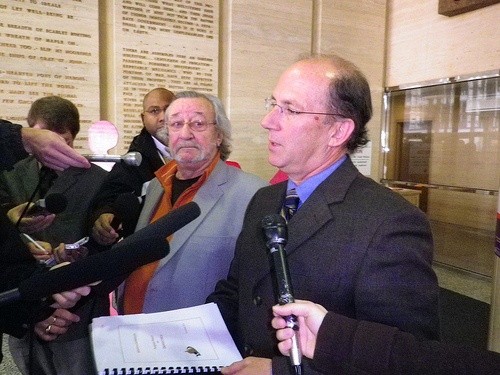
[282,184,299,222]
[42,170,57,196]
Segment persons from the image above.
[90,88,175,259]
[117,90,272,316]
[205,53,446,375]
[272,299,500,375]
[0,96,112,375]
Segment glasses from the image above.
[265,97,349,121]
[168,119,216,132]
[145,108,166,115]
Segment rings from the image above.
[45,325,52,332]
[52,316,57,324]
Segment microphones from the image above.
[83,152,143,166]
[0,236,171,307]
[115,201,202,249]
[261,214,303,375]
[28,194,67,219]
